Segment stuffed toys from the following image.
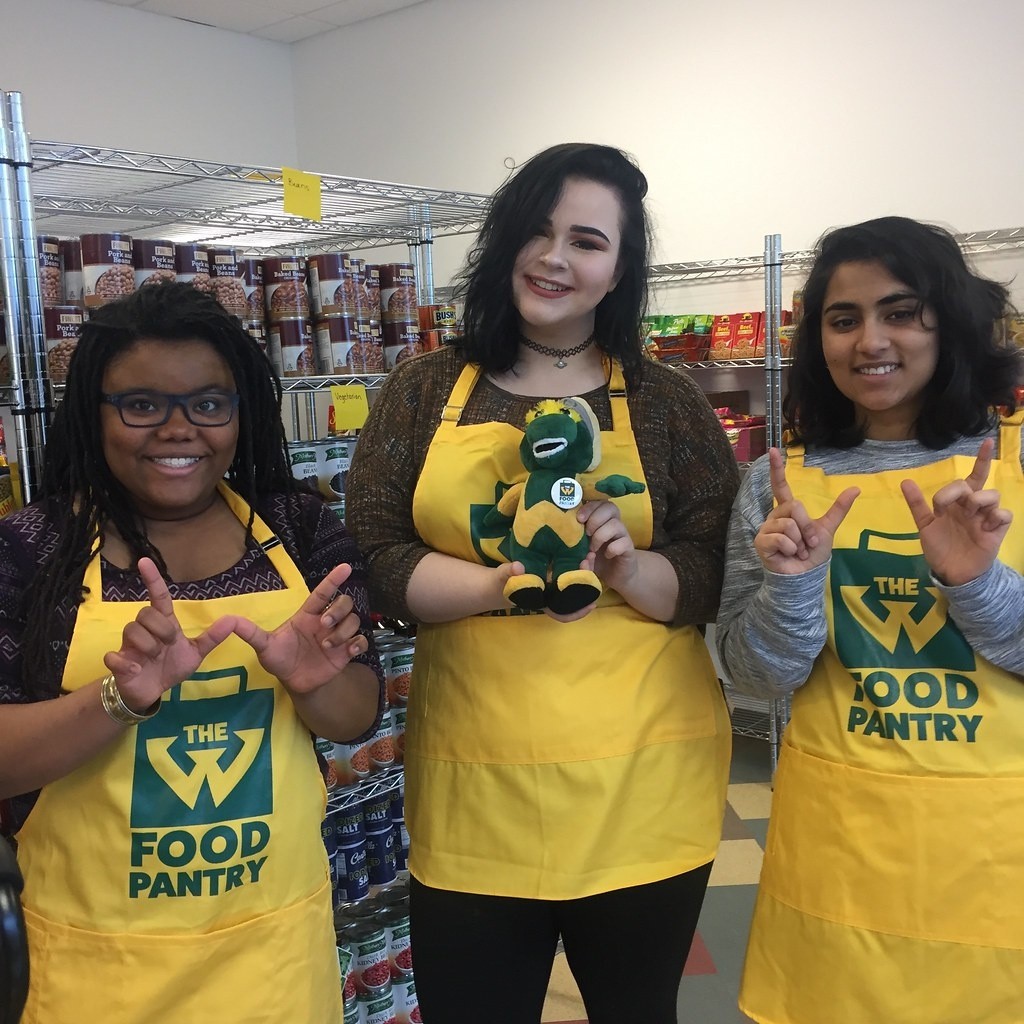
[482,395,646,615]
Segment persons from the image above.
[713,216,1024,1023]
[0,279,388,1024]
[343,142,740,1024]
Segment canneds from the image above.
[36,232,422,1024]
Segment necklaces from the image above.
[519,333,597,371]
[126,487,219,522]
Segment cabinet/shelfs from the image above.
[7,89,497,812]
[772,227,1024,449]
[438,234,774,469]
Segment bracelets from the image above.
[100,671,162,728]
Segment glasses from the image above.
[100,387,239,428]
